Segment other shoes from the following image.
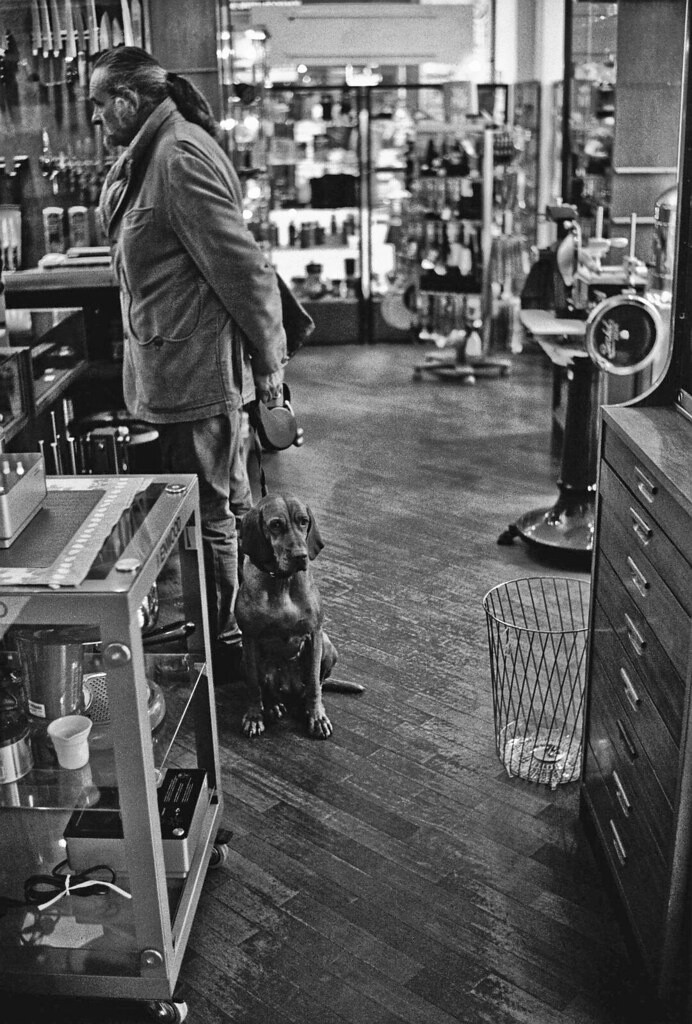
[154,647,243,685]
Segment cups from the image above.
[47,715,93,769]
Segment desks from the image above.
[521,310,593,488]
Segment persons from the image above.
[88,42,316,643]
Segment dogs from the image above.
[232,494,367,738]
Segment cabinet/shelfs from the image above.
[579,407,692,999]
[0,473,231,1024]
[223,79,510,341]
